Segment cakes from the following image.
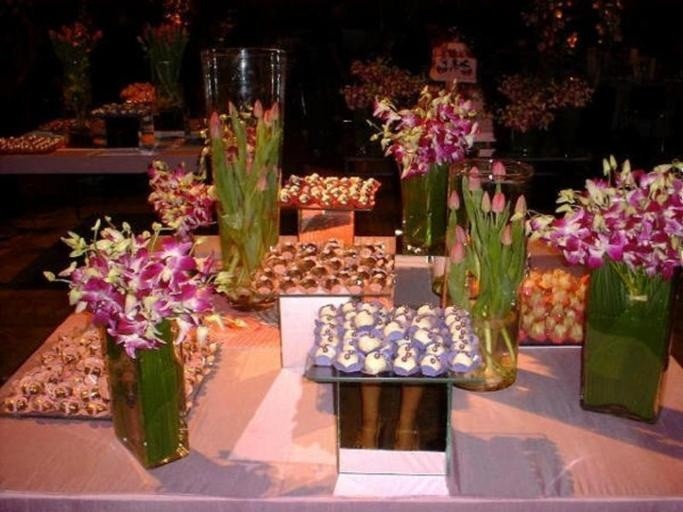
[313,300,481,374]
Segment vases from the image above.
[575,260,678,426]
[72,98,88,136]
[154,58,182,105]
[98,320,191,471]
[199,43,289,312]
[396,162,449,257]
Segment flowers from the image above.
[206,95,284,270]
[48,21,103,129]
[363,77,480,182]
[520,153,683,283]
[42,213,256,362]
[135,11,191,99]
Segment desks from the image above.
[2,210,683,512]
[0,140,204,293]
